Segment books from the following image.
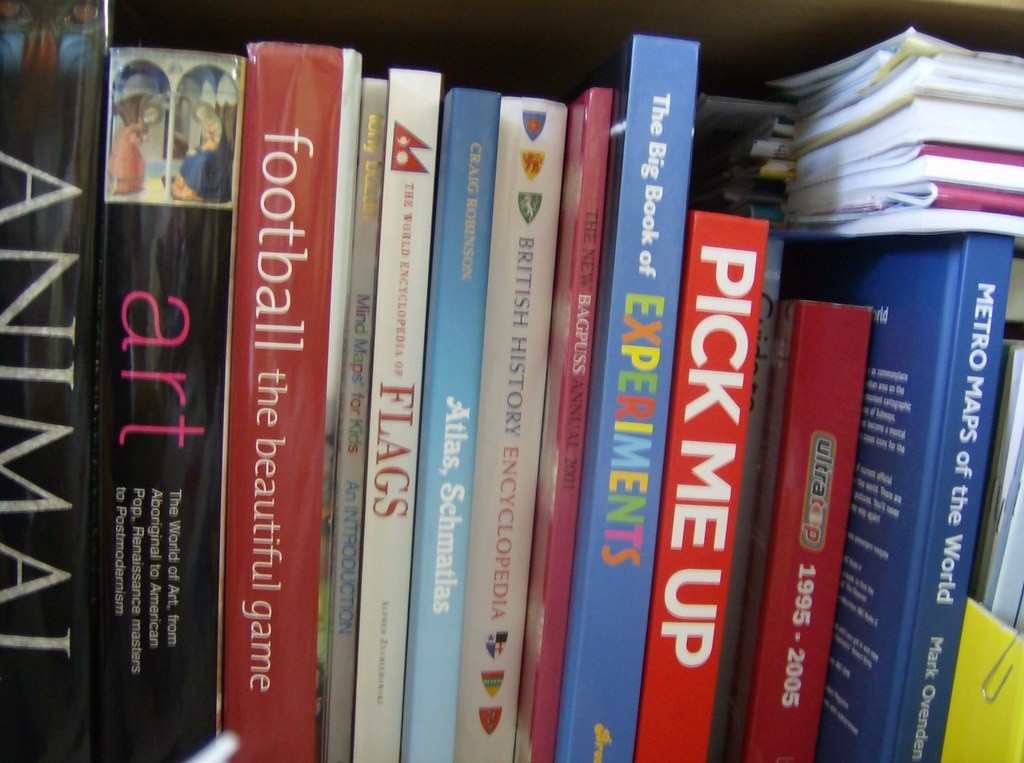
[0,0,1024,763]
